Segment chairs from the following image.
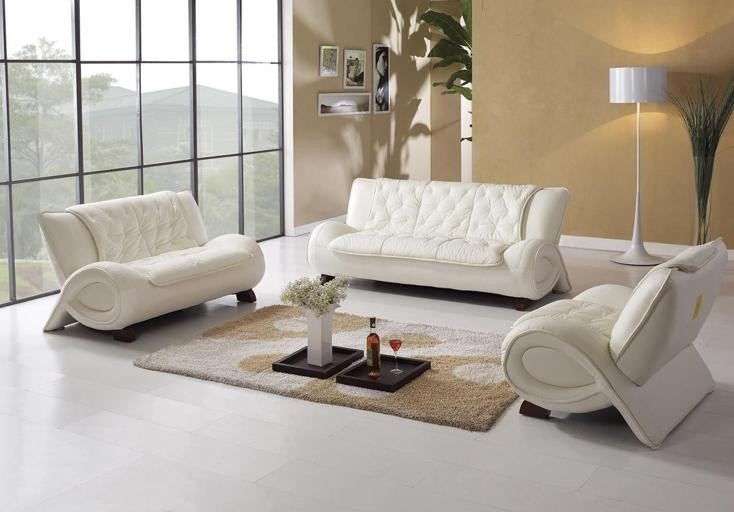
[500,235,729,451]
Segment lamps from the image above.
[606,64,671,266]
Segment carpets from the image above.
[131,302,521,434]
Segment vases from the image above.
[301,307,334,368]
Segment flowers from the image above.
[278,270,350,319]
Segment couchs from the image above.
[305,172,572,312]
[38,189,267,345]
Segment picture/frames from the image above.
[316,42,391,117]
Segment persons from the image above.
[375,47,388,111]
[349,57,360,80]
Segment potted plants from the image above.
[648,70,734,245]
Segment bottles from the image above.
[366,317,380,379]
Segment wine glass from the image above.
[387,336,405,375]
